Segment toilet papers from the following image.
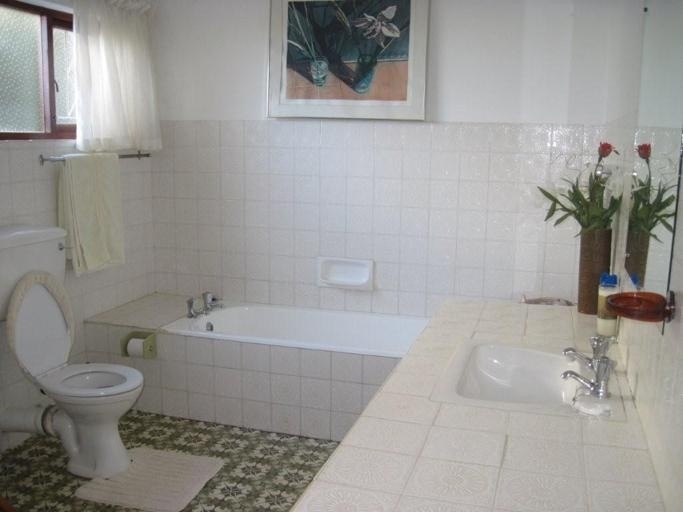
[127,337,147,360]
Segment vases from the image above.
[623,231,650,290]
[576,229,613,312]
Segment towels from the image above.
[58,151,128,274]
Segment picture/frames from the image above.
[267,1,430,124]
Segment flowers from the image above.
[629,143,678,239]
[536,139,625,234]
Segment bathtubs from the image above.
[161,305,430,445]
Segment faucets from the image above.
[202,292,223,310]
[563,336,617,368]
[186,298,209,318]
[560,356,616,398]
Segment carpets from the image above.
[72,449,225,512]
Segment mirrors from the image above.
[614,125,683,336]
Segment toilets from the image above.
[0,223,143,480]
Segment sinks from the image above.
[430,339,609,418]
